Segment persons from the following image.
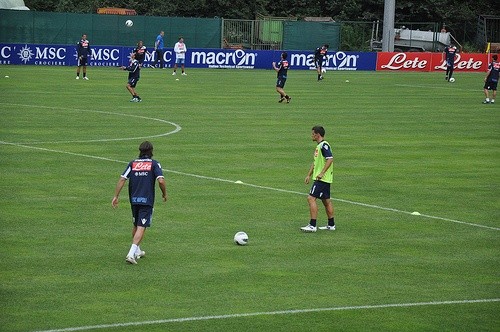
[273,52,292,103]
[112,141,168,264]
[150,31,167,70]
[133,41,147,70]
[315,44,329,81]
[443,44,457,82]
[120,52,142,103]
[300,125,337,231]
[482,55,500,104]
[76,34,92,81]
[172,36,188,76]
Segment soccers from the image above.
[126,20,133,27]
[234,232,248,245]
[321,69,326,73]
[450,78,455,82]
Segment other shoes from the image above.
[482,100,490,104]
[448,79,450,82]
[287,97,291,103]
[445,73,448,80]
[172,72,177,76]
[181,72,187,76]
[135,251,145,259]
[300,223,317,232]
[125,255,137,265]
[129,97,136,102]
[279,97,285,103]
[83,77,89,79]
[76,76,80,79]
[491,101,495,104]
[319,225,336,230]
[136,98,142,102]
